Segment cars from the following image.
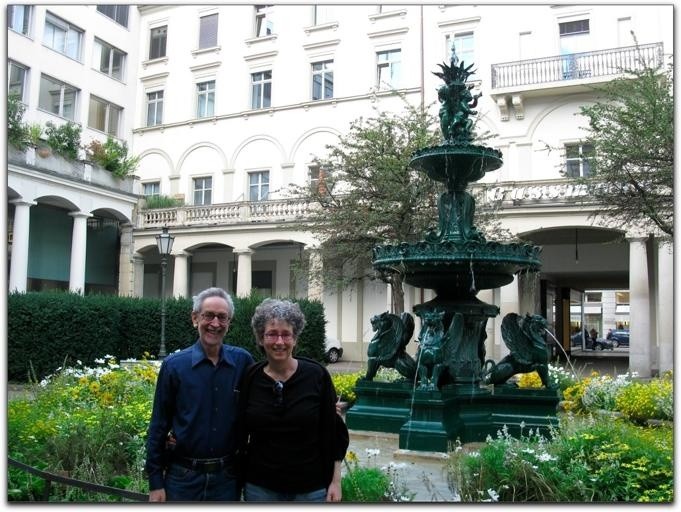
[324,335,344,363]
[586,338,614,351]
[608,328,630,347]
[570,327,588,347]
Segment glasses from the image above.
[199,312,231,323]
[272,382,284,407]
[264,332,293,340]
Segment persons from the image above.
[143,288,346,505]
[590,328,599,350]
[167,296,349,503]
[437,83,482,146]
[606,329,612,339]
[585,328,589,349]
[618,323,624,329]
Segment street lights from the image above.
[156,222,175,359]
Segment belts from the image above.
[176,455,231,473]
